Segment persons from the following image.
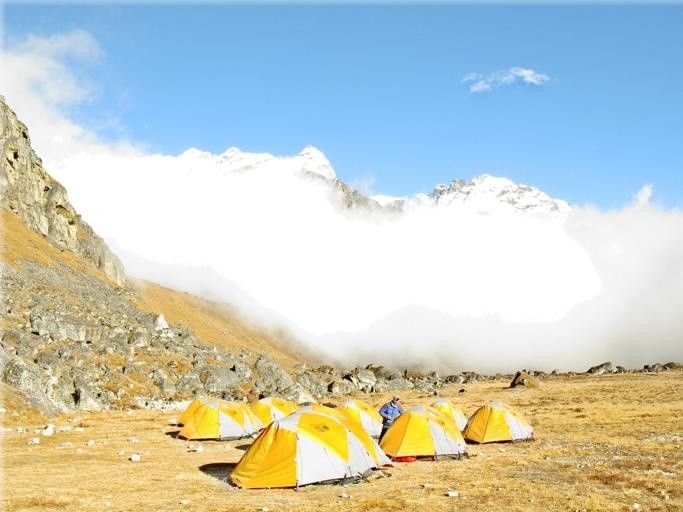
[379,395,404,445]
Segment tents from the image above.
[378,399,534,461]
[174,394,391,490]
